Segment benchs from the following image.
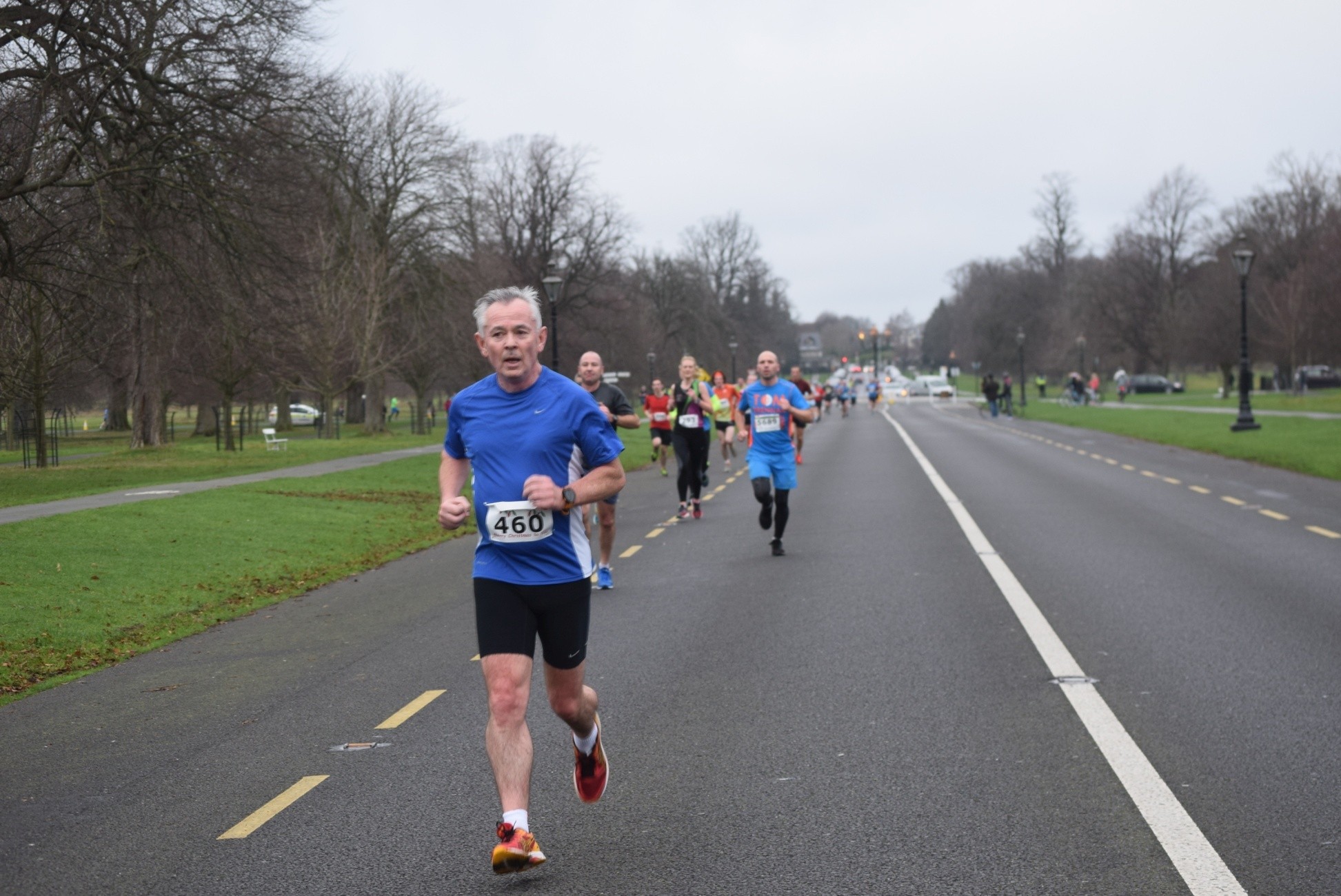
[262,427,288,452]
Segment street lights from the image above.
[1015,326,1029,406]
[645,352,659,387]
[1231,232,1266,433]
[728,335,738,385]
[857,325,892,383]
[1075,336,1087,371]
[541,260,563,373]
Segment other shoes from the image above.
[662,470,667,476]
[651,452,657,460]
[797,455,802,463]
[725,460,731,472]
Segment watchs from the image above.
[562,486,575,515]
[611,414,617,424]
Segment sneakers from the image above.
[571,712,610,804]
[676,506,689,518]
[591,558,596,572]
[692,499,702,518]
[491,820,546,874]
[759,497,773,529]
[597,567,614,589]
[770,539,785,555]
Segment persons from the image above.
[1300,367,1309,392]
[100,408,108,430]
[664,366,759,485]
[710,370,744,469]
[1062,366,1131,405]
[788,365,814,464]
[735,350,814,556]
[436,283,628,874]
[578,350,641,590]
[643,378,672,477]
[639,385,647,405]
[1035,374,1047,397]
[982,371,1014,417]
[573,372,604,525]
[667,355,714,520]
[808,377,882,422]
[388,394,400,422]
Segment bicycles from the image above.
[981,393,1026,418]
[1058,385,1095,410]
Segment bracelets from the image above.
[694,398,700,405]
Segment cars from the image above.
[1129,372,1184,394]
[826,363,874,388]
[269,404,329,428]
[884,366,954,398]
[1293,364,1341,390]
